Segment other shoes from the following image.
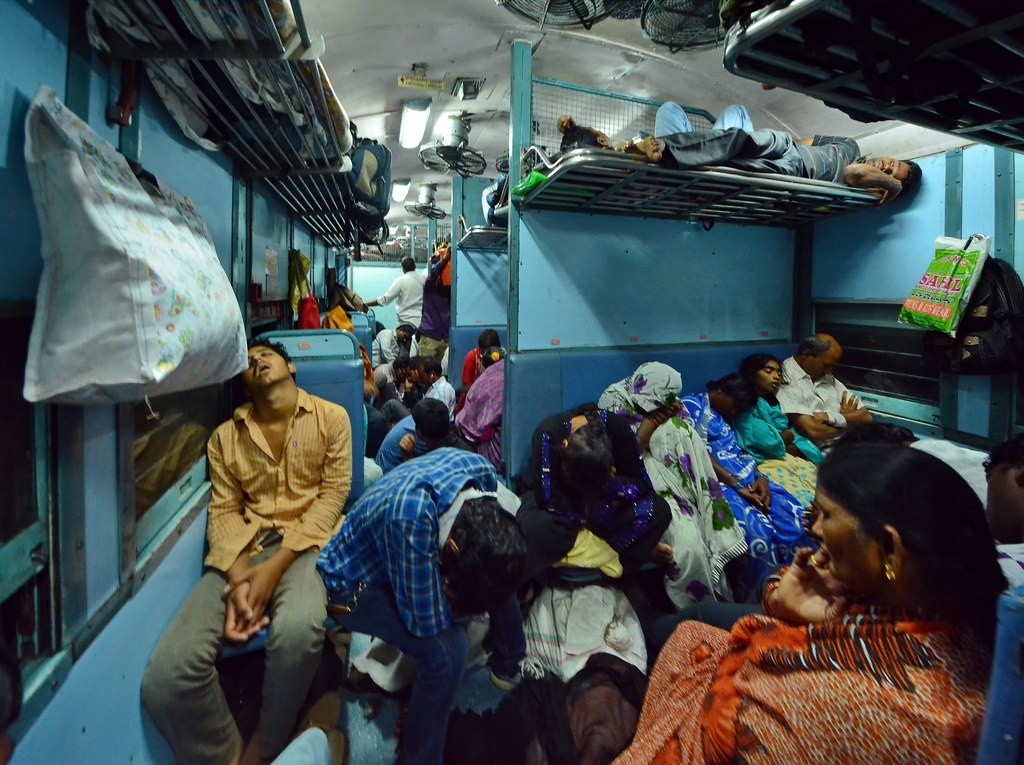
[490,668,521,690]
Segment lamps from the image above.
[496,0,728,54]
[398,100,431,149]
[392,179,411,202]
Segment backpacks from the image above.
[917,239,1024,375]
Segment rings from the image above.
[811,555,822,568]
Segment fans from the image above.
[403,184,446,219]
[495,150,510,173]
[418,117,487,177]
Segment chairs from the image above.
[364,308,377,339]
[317,309,375,404]
[217,328,369,665]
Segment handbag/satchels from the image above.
[298,296,320,329]
[286,138,389,245]
[292,250,312,315]
[897,236,992,338]
[22,85,250,406]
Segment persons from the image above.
[316,245,1024,765]
[487,102,923,209]
[141,339,353,765]
[132,397,210,527]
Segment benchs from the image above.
[506,340,802,495]
[449,324,509,395]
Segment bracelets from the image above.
[733,481,746,489]
[721,473,733,483]
[754,472,769,481]
